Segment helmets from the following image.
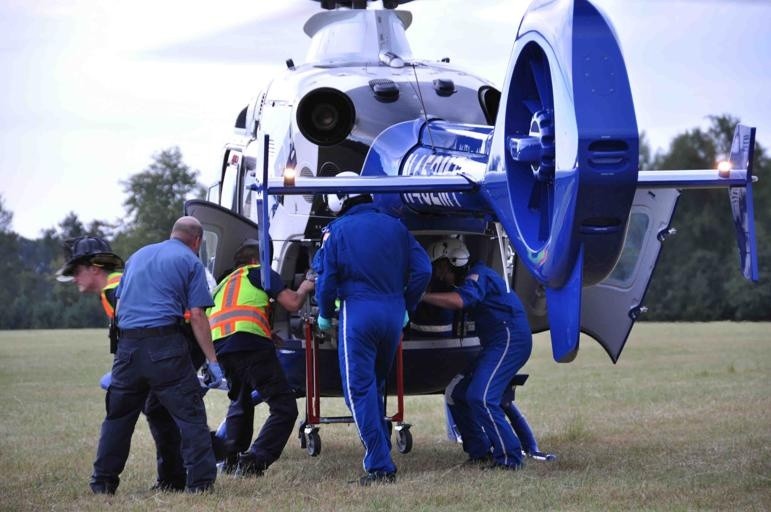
[61,236,125,275]
[322,171,370,213]
[426,239,470,267]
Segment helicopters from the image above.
[182,1,760,394]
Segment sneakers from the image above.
[357,472,396,485]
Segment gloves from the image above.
[207,362,222,388]
[317,313,333,330]
[403,310,410,327]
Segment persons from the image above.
[204,244,315,479]
[311,171,432,488]
[91,216,223,496]
[404,239,557,471]
[62,234,187,493]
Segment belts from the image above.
[117,324,180,336]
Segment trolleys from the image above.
[292,310,414,457]
